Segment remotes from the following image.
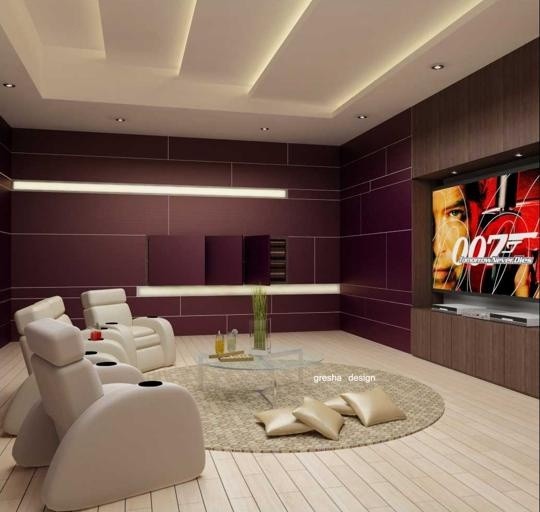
[218,354,253,361]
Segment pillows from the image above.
[291,396,345,441]
[340,385,407,426]
[323,397,356,416]
[253,408,313,436]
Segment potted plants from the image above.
[247,281,273,355]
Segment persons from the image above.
[432,184,471,290]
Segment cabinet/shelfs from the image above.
[410,306,539,398]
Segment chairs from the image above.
[3,296,129,435]
[80,288,175,374]
[12,318,206,511]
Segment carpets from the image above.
[144,360,444,453]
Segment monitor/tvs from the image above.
[431,166,540,303]
